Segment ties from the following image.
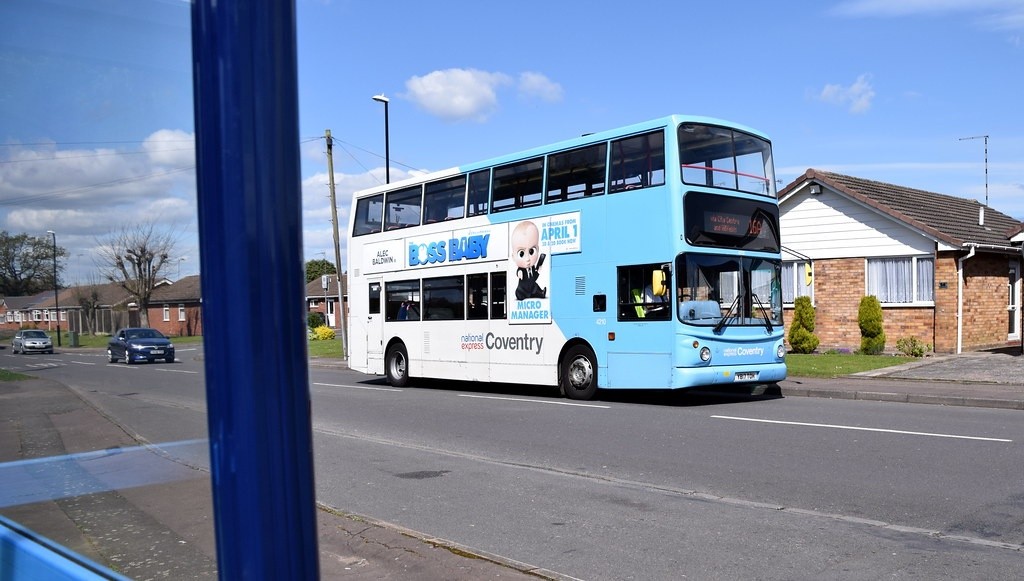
[660,295,665,303]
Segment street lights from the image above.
[47,230,61,346]
[371,93,393,185]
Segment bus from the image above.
[346,112,816,403]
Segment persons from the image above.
[640,276,672,321]
[397,302,420,320]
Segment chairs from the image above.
[406,304,420,319]
[631,288,646,318]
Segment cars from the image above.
[108,327,176,365]
[11,330,55,355]
